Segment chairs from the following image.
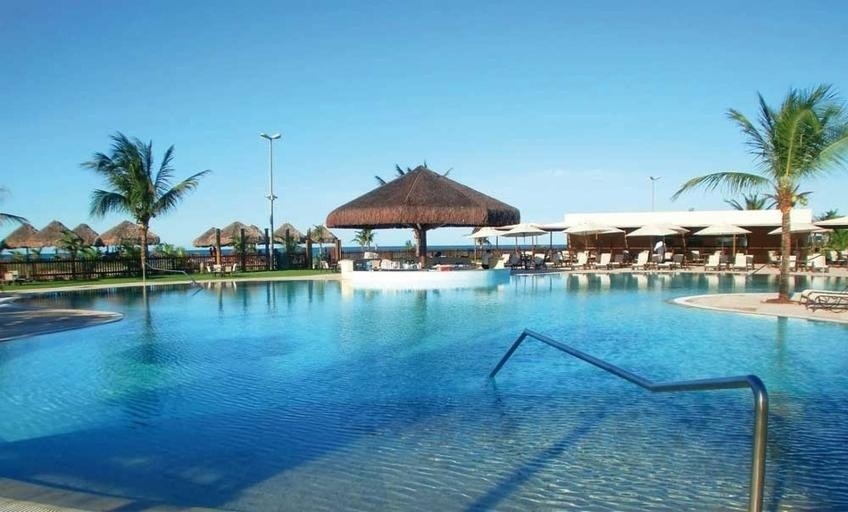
[562,273,848,293]
[2,270,25,285]
[205,261,239,276]
[318,249,848,273]
[204,282,237,294]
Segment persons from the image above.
[654,238,666,251]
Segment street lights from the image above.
[260,131,282,271]
[647,174,662,211]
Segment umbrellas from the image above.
[0,220,162,262]
[192,222,339,256]
